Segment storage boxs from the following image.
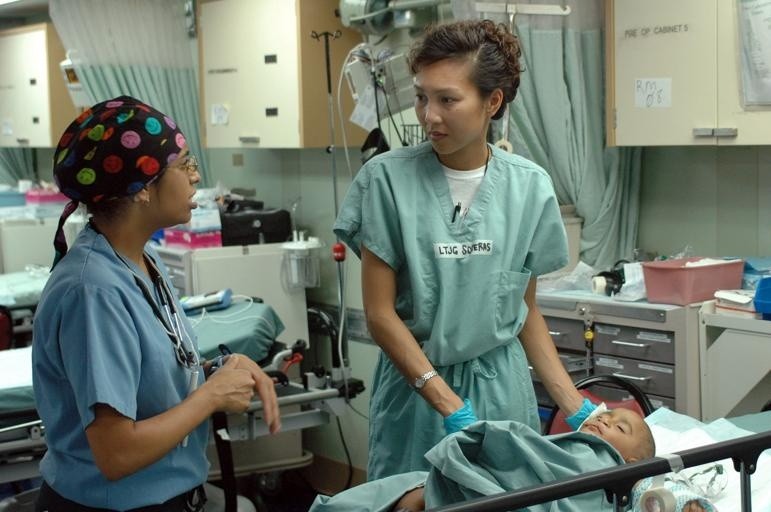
[641,256,744,307]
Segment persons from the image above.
[332,19,609,479]
[31,94,279,509]
[389,408,709,512]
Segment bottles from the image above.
[282,242,319,288]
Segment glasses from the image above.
[167,155,198,172]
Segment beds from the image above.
[312,373,771,511]
[0,265,364,512]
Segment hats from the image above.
[53,96,185,203]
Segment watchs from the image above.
[411,369,437,392]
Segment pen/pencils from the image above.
[183,369,199,447]
[452,200,462,222]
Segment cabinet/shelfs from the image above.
[155,241,315,492]
[603,1,769,149]
[2,30,83,148]
[533,313,676,418]
[199,1,370,148]
